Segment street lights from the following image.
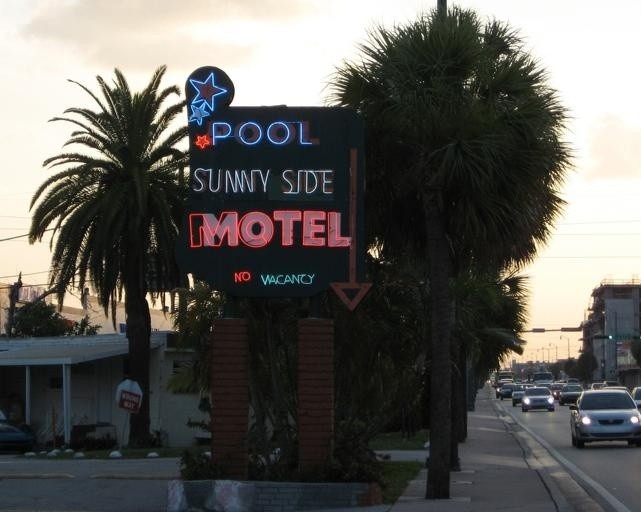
[529,335,571,365]
[601,310,618,369]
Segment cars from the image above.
[569,388,641,448]
[491,367,641,412]
[0,418,38,456]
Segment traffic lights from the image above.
[593,335,612,340]
